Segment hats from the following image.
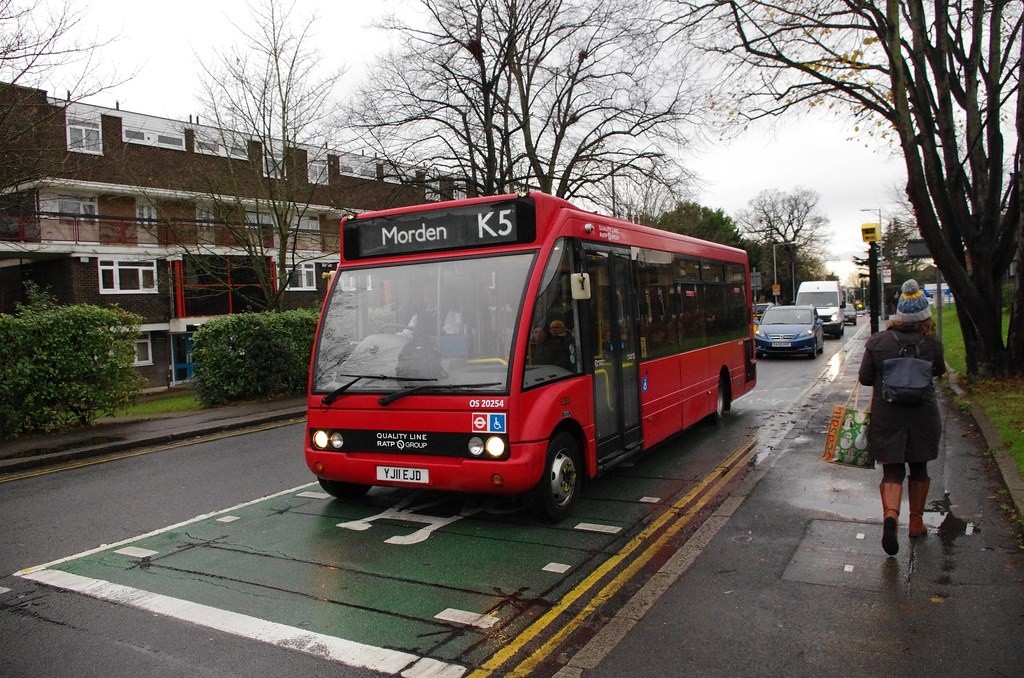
[896,279,933,323]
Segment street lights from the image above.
[861,222,882,337]
[773,241,798,306]
[860,208,886,321]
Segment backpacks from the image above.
[882,330,933,403]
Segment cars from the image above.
[843,304,858,328]
[753,305,828,359]
[752,302,774,321]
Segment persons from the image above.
[608,306,748,351]
[398,284,469,338]
[858,290,945,557]
[531,319,578,375]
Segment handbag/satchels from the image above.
[821,377,877,469]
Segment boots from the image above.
[878,483,903,556]
[907,475,931,538]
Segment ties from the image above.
[426,310,436,319]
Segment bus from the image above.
[306,193,758,516]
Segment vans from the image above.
[796,280,847,339]
